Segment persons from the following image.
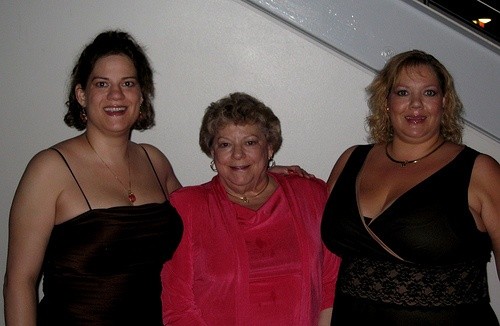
[3,30,316,326]
[160,92,342,326]
[320,49,500,326]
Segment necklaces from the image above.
[386,141,446,167]
[225,175,270,202]
[85,134,136,203]
